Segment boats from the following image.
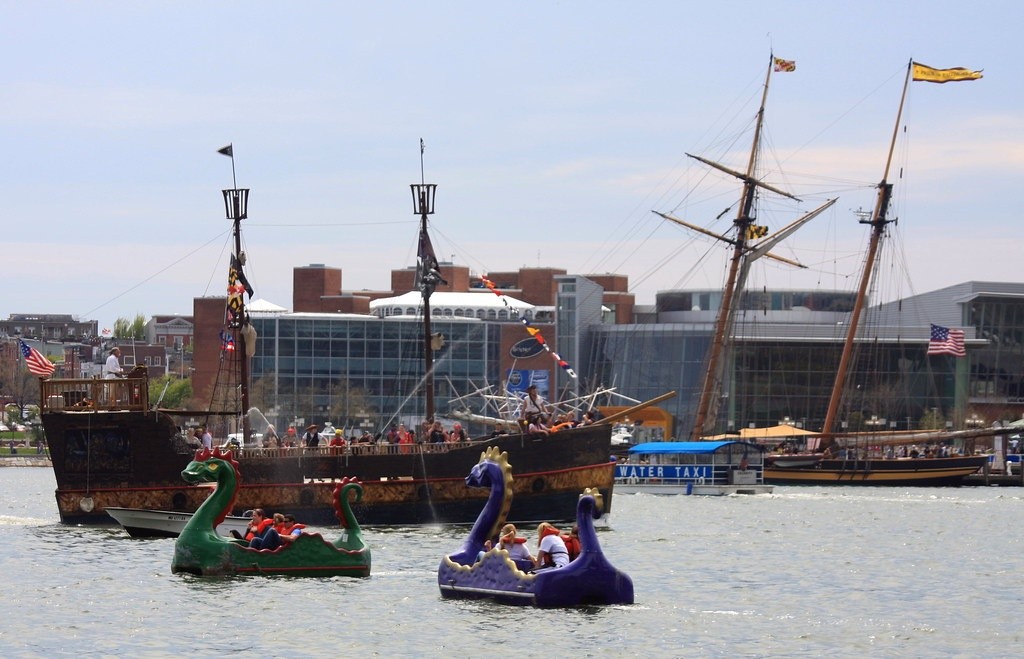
[608,439,775,498]
[168,444,373,580]
[435,443,636,610]
[38,136,621,530]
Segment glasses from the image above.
[283,520,290,523]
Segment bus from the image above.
[986,453,1024,474]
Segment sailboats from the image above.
[642,43,999,488]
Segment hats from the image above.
[304,424,319,430]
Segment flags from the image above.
[414,228,440,298]
[102,328,111,334]
[19,338,55,377]
[774,58,796,72]
[219,254,253,350]
[928,323,966,356]
[483,279,578,378]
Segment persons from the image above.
[176,423,212,451]
[37,441,43,454]
[106,347,123,411]
[473,522,580,572]
[839,441,949,458]
[230,508,305,550]
[777,440,798,454]
[522,386,595,432]
[261,421,467,454]
[10,440,14,450]
[620,457,650,484]
[491,423,506,438]
